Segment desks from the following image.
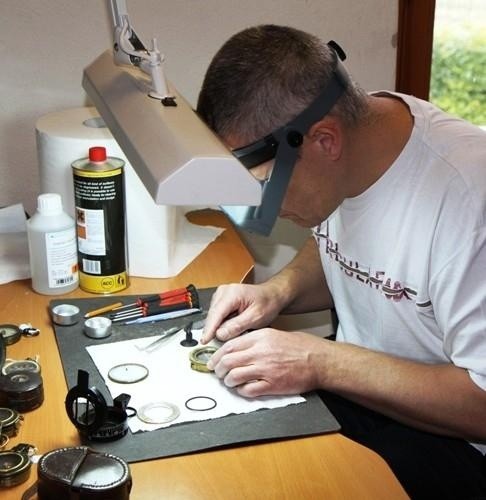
[0,202,412,500]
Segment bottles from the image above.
[73,145,131,294]
[26,191,80,295]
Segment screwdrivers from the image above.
[109,284,199,322]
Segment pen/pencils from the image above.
[84,302,123,318]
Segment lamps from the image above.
[80,0,265,207]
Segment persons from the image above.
[196,23,486,500]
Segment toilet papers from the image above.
[33,106,227,279]
